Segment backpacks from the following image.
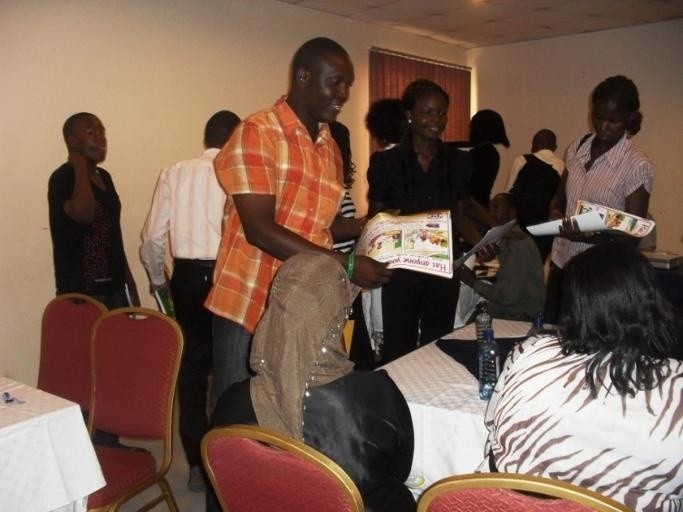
[508,154,560,232]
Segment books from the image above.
[527,200,656,239]
[350,208,454,279]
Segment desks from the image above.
[0,376,106,511]
[372,318,556,502]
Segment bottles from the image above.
[478,328,500,401]
[526,317,546,338]
[475,301,492,342]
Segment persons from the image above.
[363,98,405,217]
[328,120,375,370]
[207,250,417,512]
[543,74,654,334]
[476,244,683,512]
[368,77,461,366]
[453,194,545,323]
[468,109,512,199]
[204,37,396,512]
[139,111,241,493]
[47,113,129,448]
[505,129,566,267]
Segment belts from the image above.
[173,257,217,268]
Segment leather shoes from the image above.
[188,464,208,493]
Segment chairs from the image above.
[37,292,109,414]
[87,306,185,512]
[201,425,364,512]
[417,473,633,512]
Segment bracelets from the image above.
[348,254,354,280]
